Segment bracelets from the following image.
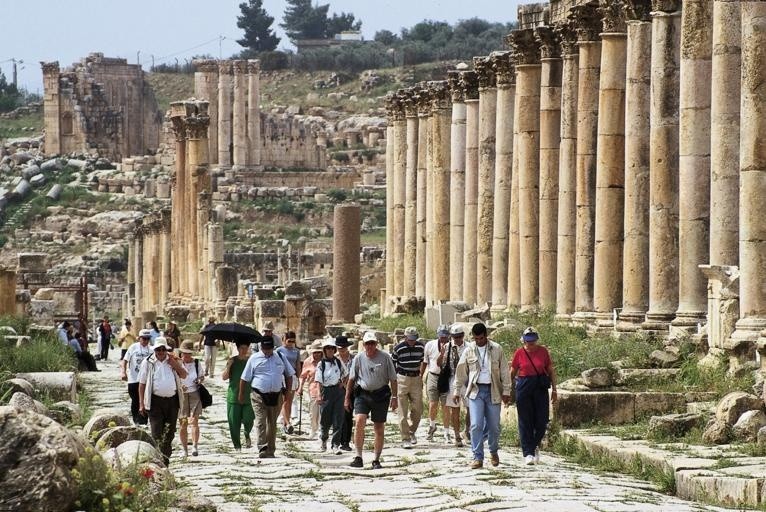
[440,353,444,356]
[391,395,398,399]
[552,390,558,393]
[419,373,423,377]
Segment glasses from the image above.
[287,340,296,343]
[154,348,167,352]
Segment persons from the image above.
[295,339,325,439]
[391,325,425,451]
[419,324,453,445]
[506,325,558,465]
[342,331,399,470]
[435,322,473,448]
[313,337,350,455]
[53,308,300,469]
[450,322,513,470]
[331,335,358,452]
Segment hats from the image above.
[308,331,378,354]
[450,325,464,337]
[178,339,198,354]
[262,337,274,350]
[436,325,450,339]
[139,328,153,337]
[167,321,177,326]
[404,326,420,341]
[206,315,216,323]
[261,320,277,330]
[150,337,172,350]
[523,327,540,342]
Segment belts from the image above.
[361,386,386,393]
[399,371,420,377]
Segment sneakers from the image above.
[180,449,188,458]
[246,437,251,446]
[350,456,364,467]
[470,459,483,469]
[410,432,417,444]
[284,424,292,433]
[526,454,534,465]
[192,446,198,456]
[535,447,540,465]
[488,451,499,466]
[372,460,382,469]
[426,428,472,446]
[402,439,412,449]
[308,431,353,455]
[292,417,300,427]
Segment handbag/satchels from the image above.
[438,368,451,392]
[261,393,280,407]
[537,372,552,389]
[197,384,213,409]
